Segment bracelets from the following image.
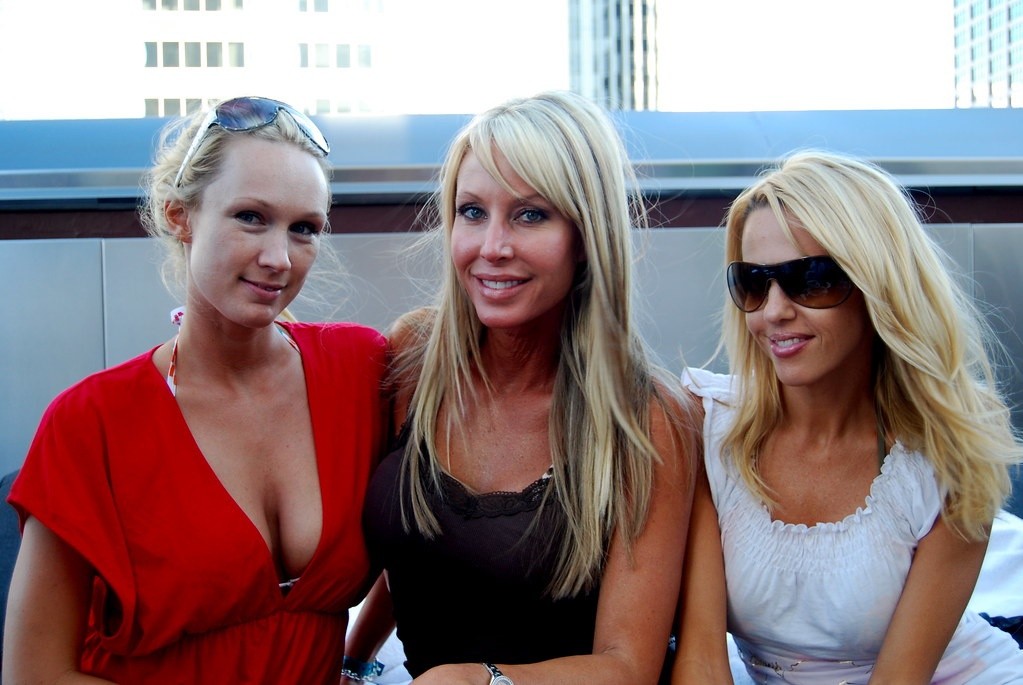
[341,655,377,681]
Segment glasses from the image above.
[177,95,330,189]
[726,255,858,313]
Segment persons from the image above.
[670,152,1023,685]
[2,106,390,684]
[341,93,696,685]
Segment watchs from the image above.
[483,662,514,685]
